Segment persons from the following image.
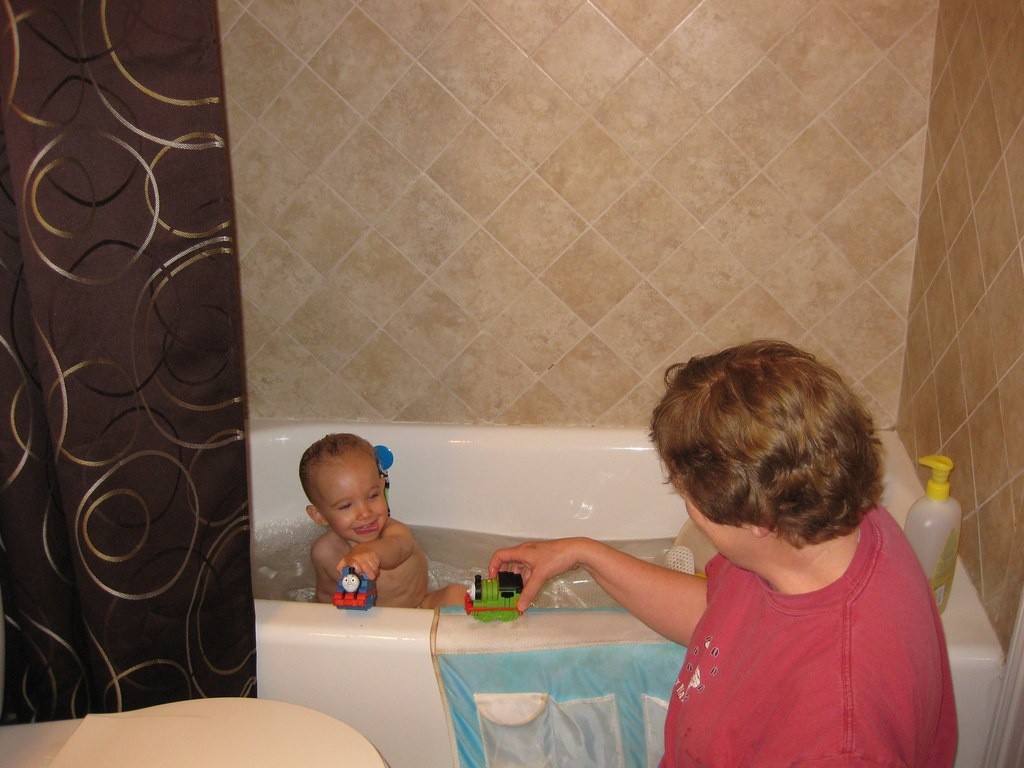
[484,338,960,767]
[298,432,471,610]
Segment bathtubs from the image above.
[247,418,1010,768]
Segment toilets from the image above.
[1,699,392,768]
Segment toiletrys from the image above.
[902,454,962,619]
